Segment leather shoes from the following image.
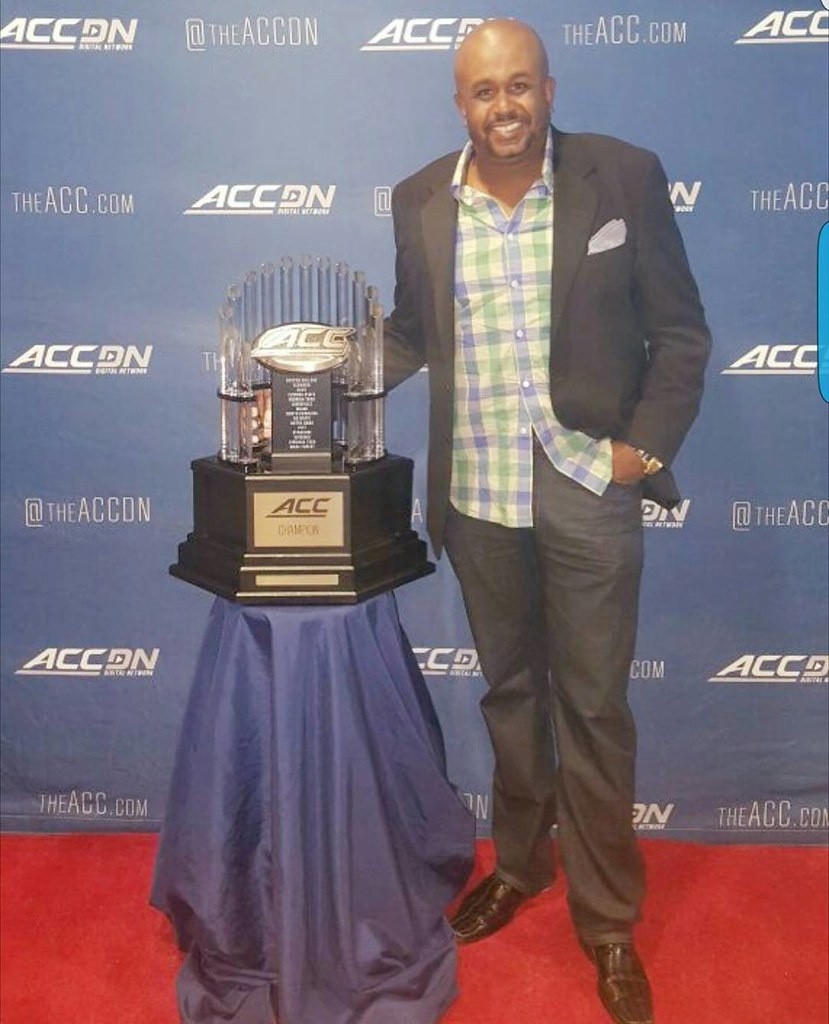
[582,940,653,1024]
[448,872,525,943]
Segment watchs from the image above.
[633,448,662,477]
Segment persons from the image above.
[239,20,711,1024]
